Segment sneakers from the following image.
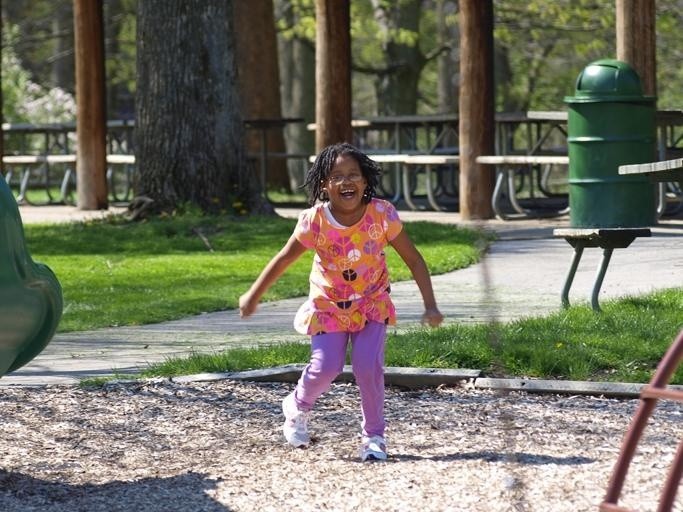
[282,393,311,448]
[360,433,387,463]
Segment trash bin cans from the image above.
[563,58,659,229]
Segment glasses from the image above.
[320,174,364,186]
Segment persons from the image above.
[237,141,444,461]
